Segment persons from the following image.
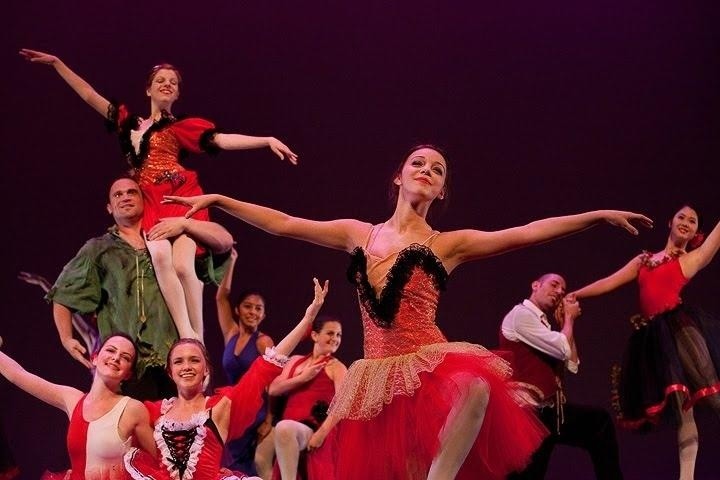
[556,202,720,480]
[499,264,622,480]
[19,47,299,339]
[159,145,654,480]
